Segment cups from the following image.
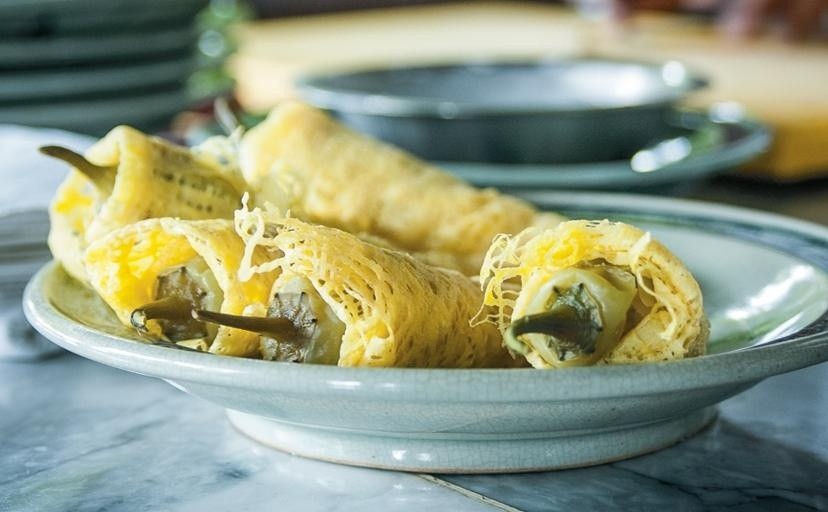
[0,123,102,360]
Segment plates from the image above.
[0,1,231,134]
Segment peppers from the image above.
[504,258,636,368]
[131,255,223,350]
[38,145,120,221]
[190,272,347,365]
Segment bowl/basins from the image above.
[36,195,823,476]
[429,106,774,196]
[297,53,709,165]
